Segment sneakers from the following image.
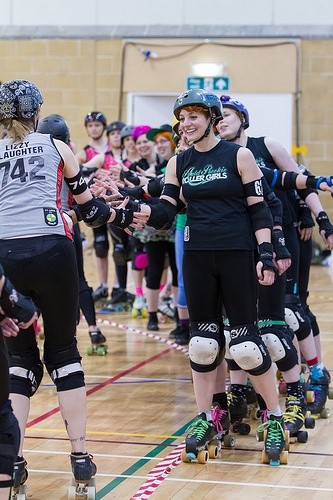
[147,316,158,331]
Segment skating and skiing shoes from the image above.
[285,397,315,443]
[87,328,108,357]
[92,287,108,313]
[67,452,98,500]
[262,414,290,465]
[228,368,333,435]
[11,455,32,500]
[131,297,148,318]
[157,296,176,324]
[210,402,236,447]
[181,412,219,464]
[103,288,129,313]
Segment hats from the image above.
[147,123,172,141]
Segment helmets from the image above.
[84,111,106,126]
[120,125,134,142]
[172,116,180,136]
[108,122,126,135]
[37,114,70,143]
[0,79,44,121]
[174,88,224,124]
[132,125,152,142]
[219,95,249,130]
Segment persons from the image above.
[38,90,333,465]
[0,80,149,500]
[0,264,41,500]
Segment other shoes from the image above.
[170,329,181,338]
[176,336,190,344]
